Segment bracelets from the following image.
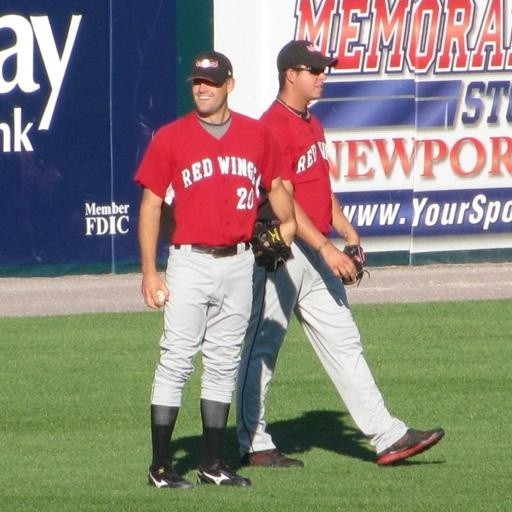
[315,239,331,251]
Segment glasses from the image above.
[290,67,325,76]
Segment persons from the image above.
[237,39,445,469]
[131,52,302,492]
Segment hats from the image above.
[277,40,338,72]
[188,52,232,87]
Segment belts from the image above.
[175,242,251,258]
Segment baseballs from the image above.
[157,290,165,304]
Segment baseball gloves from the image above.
[253,217,291,271]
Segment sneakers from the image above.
[197,463,252,486]
[376,428,444,465]
[240,449,304,468]
[148,466,194,489]
